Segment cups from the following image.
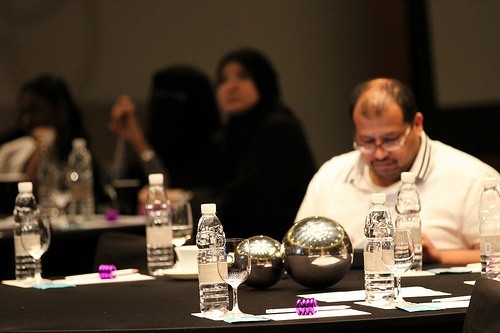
[170,202,194,247]
[173,245,199,272]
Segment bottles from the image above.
[394,171,423,275]
[67,139,95,226]
[194,203,229,316]
[364,193,396,310]
[12,181,42,285]
[143,173,174,275]
[478,177,500,281]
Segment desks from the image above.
[0,215,148,280]
[0,263,500,333]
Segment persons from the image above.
[294,78,500,266]
[0,48,316,248]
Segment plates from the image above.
[160,268,199,279]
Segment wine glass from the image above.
[19,215,52,286]
[217,238,256,318]
[382,228,419,307]
[53,170,78,229]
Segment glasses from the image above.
[353,125,413,155]
